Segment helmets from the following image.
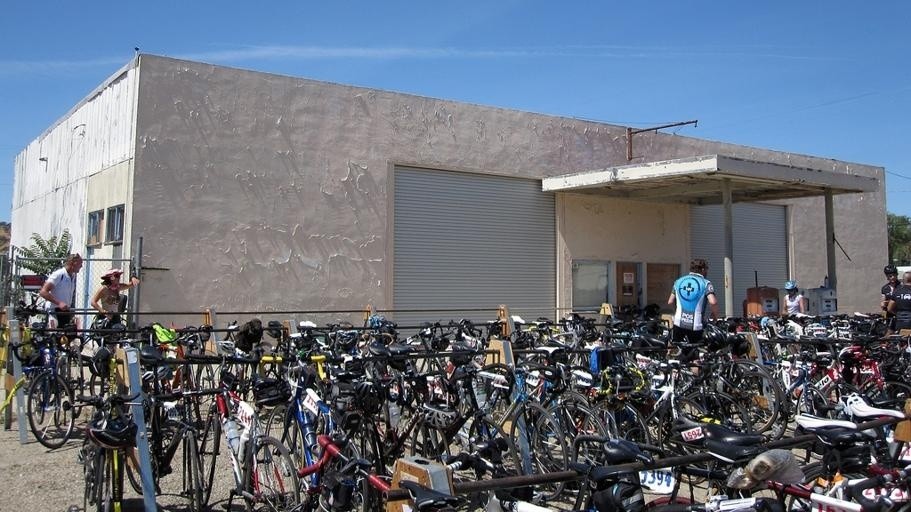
[784,281,797,290]
[884,265,897,274]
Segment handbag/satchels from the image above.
[89,318,111,335]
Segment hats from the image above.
[101,269,123,279]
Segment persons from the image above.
[39,252,83,367]
[782,280,805,315]
[887,272,911,329]
[880,264,903,331]
[667,259,720,346]
[90,268,139,360]
